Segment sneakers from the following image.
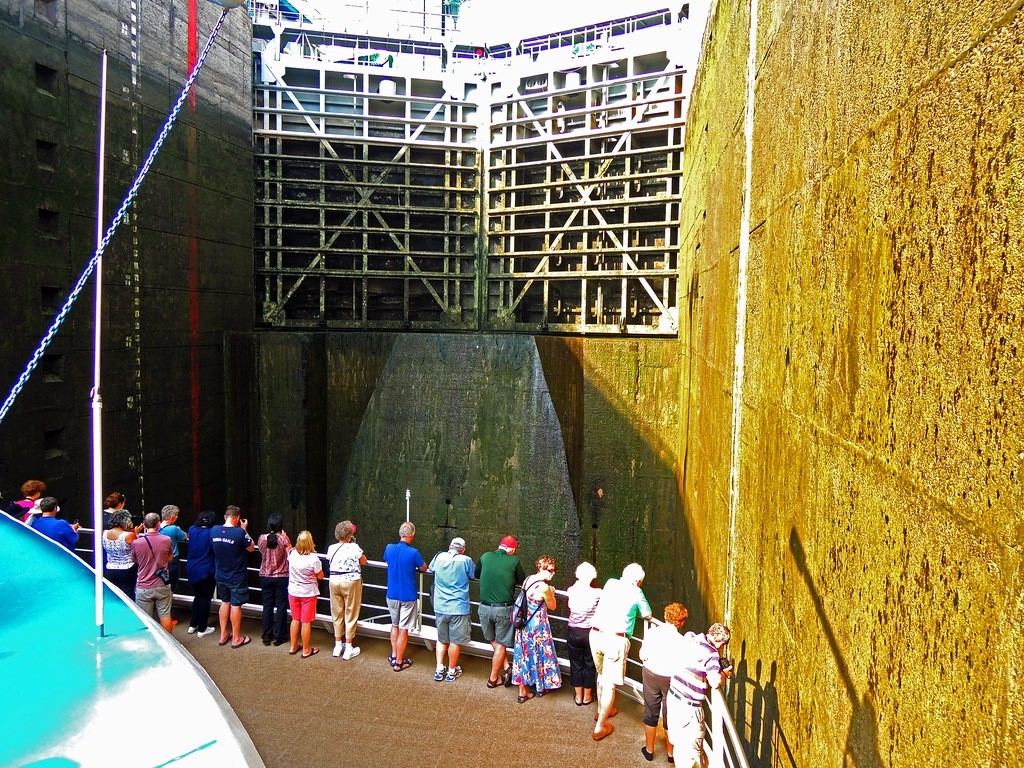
[333,642,360,660]
[445,665,462,682]
[434,666,447,680]
[187,627,215,638]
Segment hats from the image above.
[499,535,518,551]
[450,537,466,550]
[5,503,29,518]
[27,498,60,514]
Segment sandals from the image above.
[486,673,505,688]
[518,690,535,703]
[536,687,553,697]
[504,665,513,687]
[389,654,413,671]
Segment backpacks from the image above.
[509,574,545,630]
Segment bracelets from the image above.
[722,670,729,679]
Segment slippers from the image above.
[300,647,319,658]
[219,634,251,649]
[572,691,597,706]
[262,639,286,646]
[289,644,303,655]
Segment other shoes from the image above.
[666,753,672,763]
[594,705,618,722]
[641,747,653,761]
[592,723,613,741]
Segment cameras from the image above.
[720,658,733,680]
[155,569,171,585]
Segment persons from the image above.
[287,531,324,657]
[102,510,144,601]
[666,623,733,768]
[188,510,216,637]
[567,561,602,705]
[588,562,652,740]
[327,520,367,661]
[16,480,44,523]
[383,522,428,672]
[131,512,173,640]
[6,504,30,523]
[428,537,476,681]
[474,536,525,688]
[157,505,187,626]
[25,498,59,526]
[257,513,290,646]
[103,492,129,529]
[641,603,689,763]
[30,497,79,553]
[211,505,255,649]
[510,555,563,703]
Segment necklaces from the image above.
[24,498,34,501]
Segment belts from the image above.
[481,601,513,607]
[591,626,627,638]
[668,688,702,707]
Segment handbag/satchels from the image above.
[429,585,434,608]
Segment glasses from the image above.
[174,515,179,519]
[546,568,556,573]
